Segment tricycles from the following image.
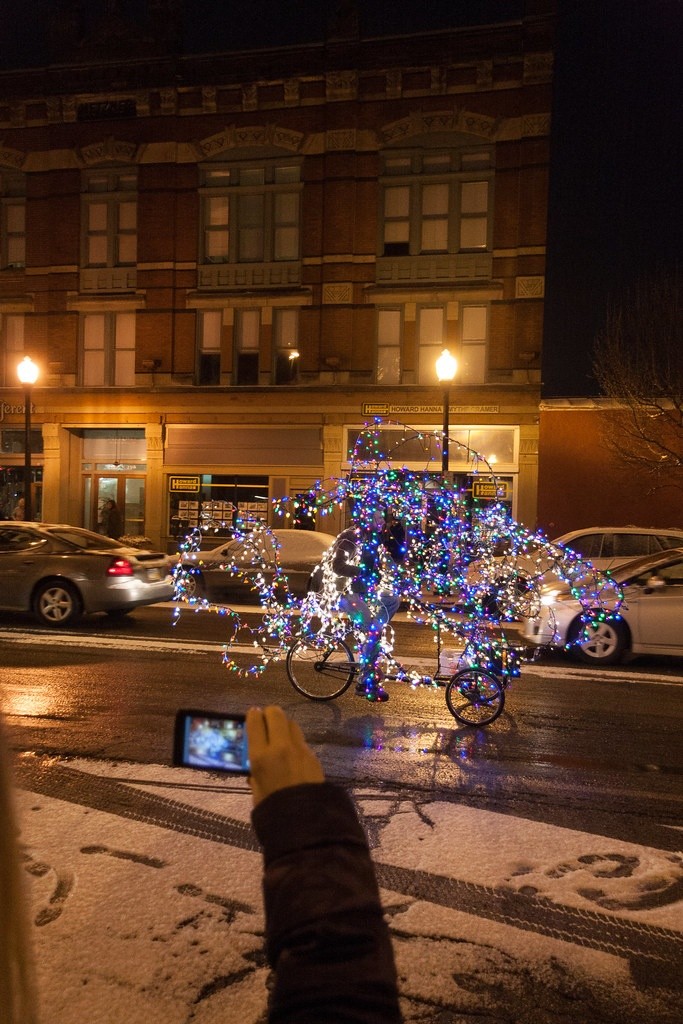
[287,581,524,726]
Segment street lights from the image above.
[16,355,41,520]
[436,349,460,593]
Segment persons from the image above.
[245,704,406,1024]
[11,497,26,521]
[306,501,423,703]
[104,500,120,540]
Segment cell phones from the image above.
[173,709,251,778]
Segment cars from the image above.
[1,521,174,629]
[174,530,338,602]
[517,548,683,668]
[464,525,683,622]
